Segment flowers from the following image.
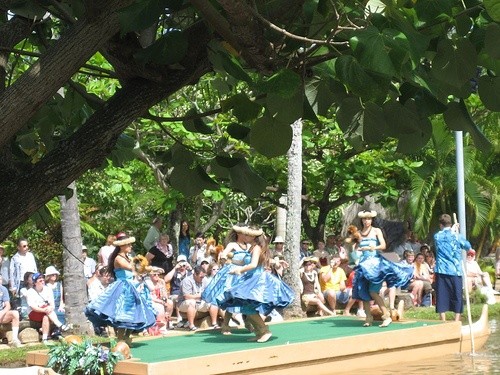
[45,334,125,375]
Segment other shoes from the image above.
[41,339,48,343]
[136,317,220,337]
[57,325,70,332]
[313,310,366,318]
[11,339,22,346]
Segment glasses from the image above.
[153,274,161,276]
[197,274,205,279]
[275,242,284,244]
[19,244,29,248]
[35,279,45,284]
[301,242,310,246]
[201,262,208,265]
[467,254,474,258]
[49,274,58,276]
[421,247,428,250]
[304,262,316,266]
[211,268,220,271]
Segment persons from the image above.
[86,232,155,347]
[81,220,286,336]
[0,239,69,347]
[350,208,413,327]
[299,234,500,320]
[434,214,475,321]
[230,225,294,344]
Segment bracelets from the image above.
[235,265,243,274]
[371,245,375,251]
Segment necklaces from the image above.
[236,242,247,251]
[249,245,255,252]
[118,252,131,263]
[359,226,372,236]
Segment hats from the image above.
[272,236,286,245]
[81,245,87,250]
[43,266,61,276]
[176,255,187,262]
[468,250,476,255]
[420,243,430,252]
[31,273,45,284]
[145,266,165,274]
